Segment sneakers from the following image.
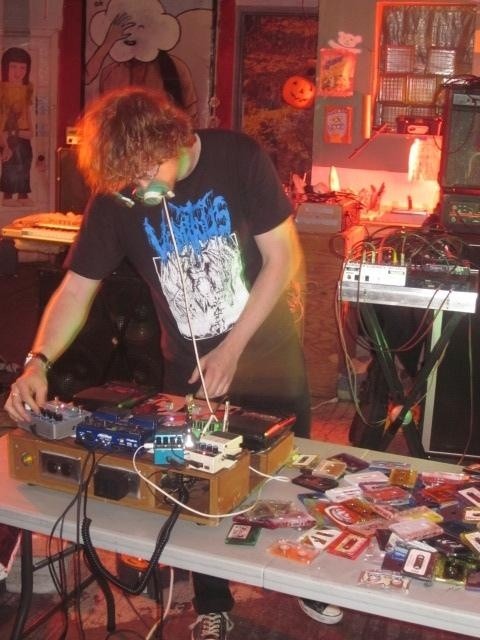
[188,611,234,640]
[298,598,345,625]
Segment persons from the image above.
[0,47,36,202]
[84,12,136,86]
[1,87,343,640]
[98,48,201,131]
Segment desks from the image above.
[0,427,480,640]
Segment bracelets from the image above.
[24,350,54,372]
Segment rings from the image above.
[12,393,20,398]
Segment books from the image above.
[34,220,80,232]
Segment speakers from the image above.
[440,106,480,189]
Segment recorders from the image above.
[397,116,442,135]
